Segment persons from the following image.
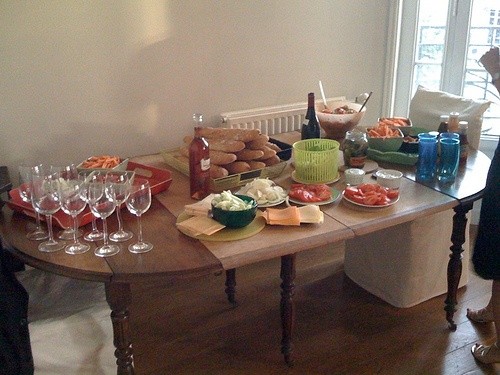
[466,47,500,364]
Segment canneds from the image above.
[342,130,368,169]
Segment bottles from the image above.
[301,93,321,141]
[188,113,211,200]
[437,111,469,164]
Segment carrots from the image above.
[369,118,407,139]
[79,155,121,169]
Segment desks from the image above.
[0,126,492,375]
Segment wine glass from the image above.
[18,160,153,257]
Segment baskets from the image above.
[161,133,293,190]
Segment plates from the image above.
[286,185,341,206]
[233,189,286,207]
[341,189,400,208]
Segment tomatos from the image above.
[288,183,331,202]
[344,184,400,205]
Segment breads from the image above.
[174,126,281,177]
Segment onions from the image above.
[212,190,253,211]
[241,177,284,205]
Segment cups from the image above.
[416,132,461,185]
[376,169,403,189]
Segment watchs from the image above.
[492,72,500,85]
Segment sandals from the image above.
[466,306,499,324]
[471,342,500,365]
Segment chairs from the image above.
[0,269,117,375]
[344,87,493,307]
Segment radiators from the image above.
[221,96,349,137]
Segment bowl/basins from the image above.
[211,195,259,229]
[315,102,366,139]
[401,136,419,154]
[365,127,405,152]
[76,155,129,175]
[378,117,413,137]
